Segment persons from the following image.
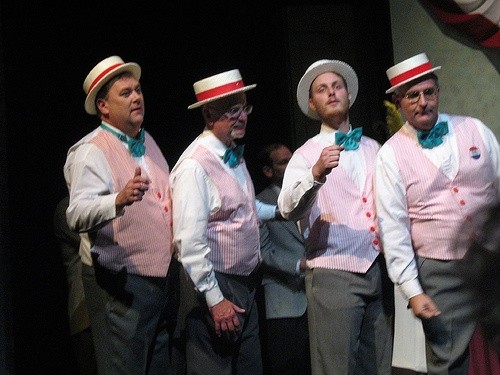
[169,52,500,375]
[62,56,178,375]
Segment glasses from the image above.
[404,89,436,103]
[224,105,253,119]
[271,159,288,171]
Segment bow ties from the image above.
[125,127,147,159]
[218,144,247,170]
[416,121,450,150]
[334,127,363,151]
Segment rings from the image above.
[132,189,136,195]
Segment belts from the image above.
[226,270,259,283]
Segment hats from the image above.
[295,58,360,120]
[81,55,143,116]
[186,69,257,110]
[384,52,442,94]
